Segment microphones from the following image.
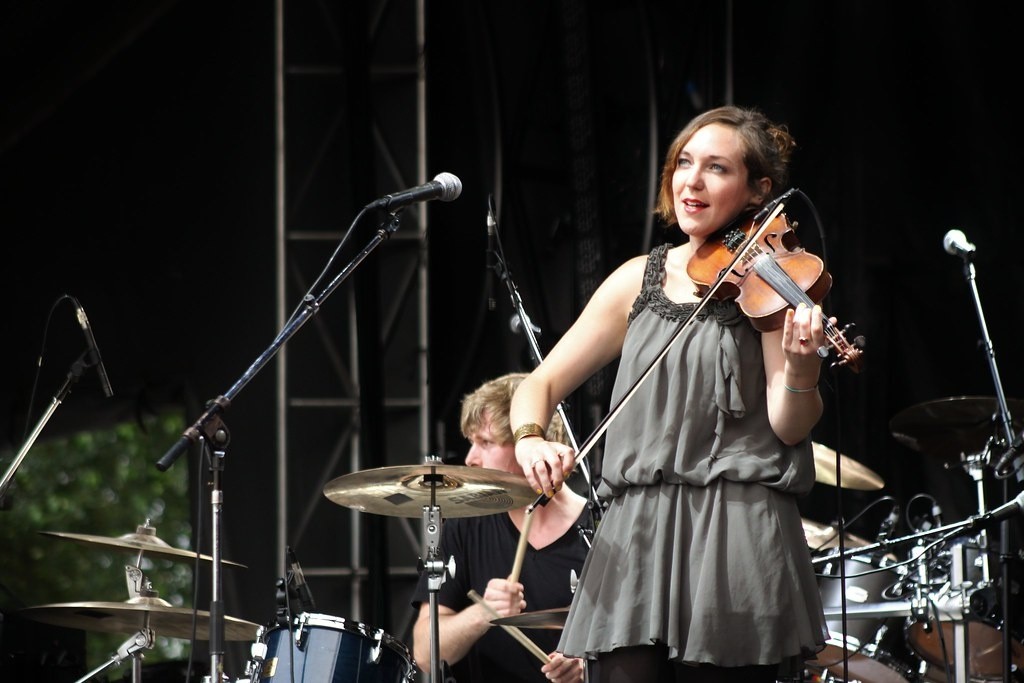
[494,262,532,337]
[364,170,462,214]
[943,228,983,267]
[753,188,796,223]
[69,293,116,398]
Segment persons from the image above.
[508,104,837,683]
[410,373,599,683]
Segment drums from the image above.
[230,610,430,683]
[808,522,1023,683]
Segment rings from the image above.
[798,337,812,346]
[531,457,544,468]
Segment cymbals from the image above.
[488,599,573,631]
[811,439,885,492]
[321,453,545,521]
[35,517,254,572]
[798,515,899,565]
[15,577,269,643]
[905,392,1019,437]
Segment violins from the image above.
[684,198,868,377]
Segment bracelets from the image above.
[783,382,819,394]
[512,424,547,448]
